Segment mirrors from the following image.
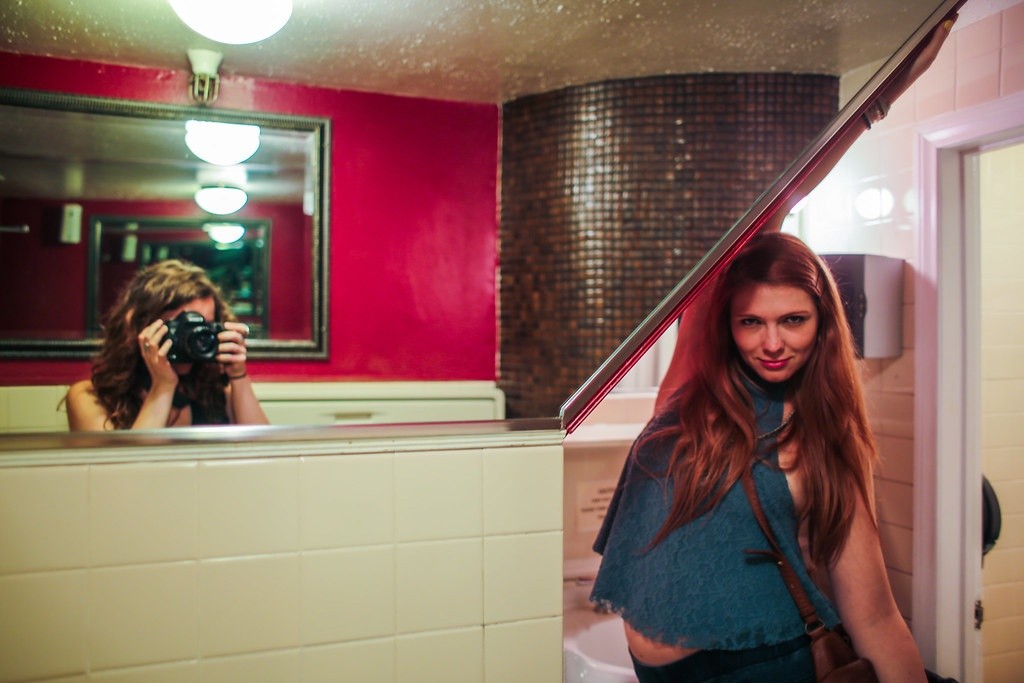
[0,89,332,361]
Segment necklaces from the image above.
[756,407,798,441]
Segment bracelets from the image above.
[228,372,246,380]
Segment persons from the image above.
[56,257,271,433]
[587,233,960,683]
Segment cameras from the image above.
[159,311,225,363]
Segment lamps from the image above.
[168,0,295,46]
[180,120,263,247]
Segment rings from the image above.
[144,342,150,351]
[244,327,250,338]
